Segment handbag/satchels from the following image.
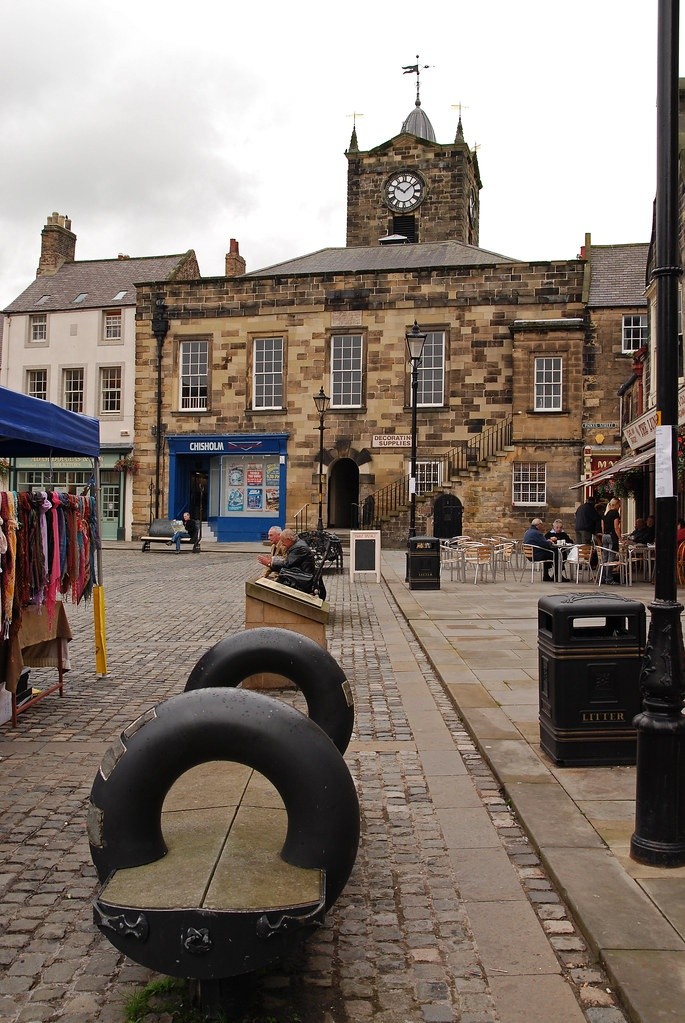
[567,545,585,562]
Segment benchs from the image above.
[87,626,361,982]
[141,517,205,552]
[277,538,340,599]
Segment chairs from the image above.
[437,535,657,585]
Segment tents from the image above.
[569,447,655,489]
[0,385,107,679]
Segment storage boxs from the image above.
[16,684,34,711]
[14,665,36,694]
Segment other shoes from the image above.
[606,580,619,585]
[552,573,570,582]
[543,576,554,582]
[175,550,180,554]
[599,580,606,584]
[166,541,172,546]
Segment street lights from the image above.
[150,297,170,519]
[404,319,428,548]
[313,386,331,532]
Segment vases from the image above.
[0,467,5,475]
[119,464,134,472]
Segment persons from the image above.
[573,496,601,570]
[166,512,198,554]
[677,518,685,547]
[621,514,655,573]
[191,475,208,521]
[544,519,575,573]
[602,497,624,584]
[523,518,570,582]
[258,526,315,593]
[590,503,603,569]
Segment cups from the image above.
[557,539,566,545]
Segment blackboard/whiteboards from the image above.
[350,530,380,573]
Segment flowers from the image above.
[114,457,140,475]
[0,460,11,476]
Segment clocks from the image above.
[382,171,425,210]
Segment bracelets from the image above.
[266,559,271,566]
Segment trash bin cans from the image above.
[538,592,647,769]
[408,536,441,591]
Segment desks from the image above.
[10,602,72,729]
[550,543,574,582]
[622,544,654,582]
